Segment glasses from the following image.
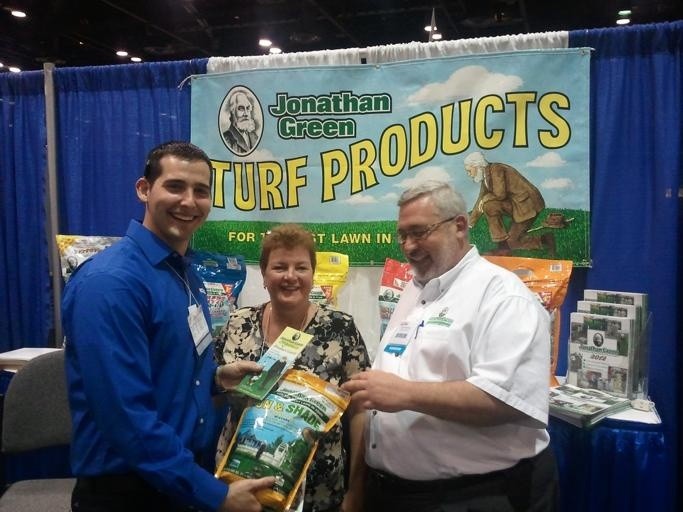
[396,214,457,244]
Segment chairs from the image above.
[0,348,77,512]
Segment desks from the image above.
[556,374,661,431]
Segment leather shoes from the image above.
[543,231,555,256]
[483,249,512,257]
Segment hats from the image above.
[541,213,569,229]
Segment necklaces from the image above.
[260,305,307,360]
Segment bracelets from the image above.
[214,364,228,396]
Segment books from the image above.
[549,284,651,433]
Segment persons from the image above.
[338,180,555,508]
[64,254,77,274]
[60,142,281,512]
[221,90,261,154]
[461,150,552,255]
[216,225,371,511]
